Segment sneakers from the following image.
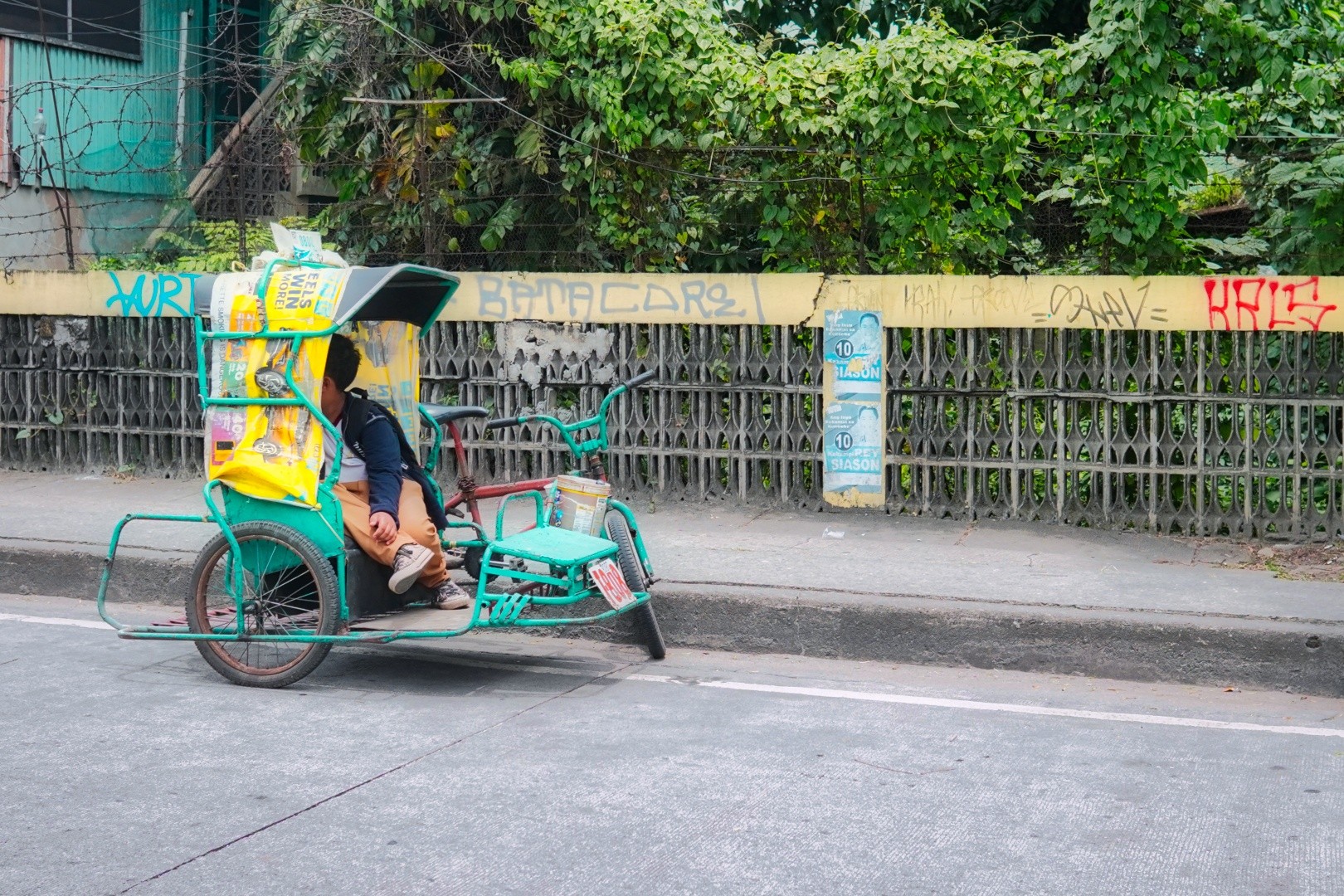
[389,543,434,594]
[431,578,470,608]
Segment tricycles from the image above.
[96,249,667,690]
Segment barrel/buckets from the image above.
[549,475,611,538]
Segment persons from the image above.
[321,332,472,610]
